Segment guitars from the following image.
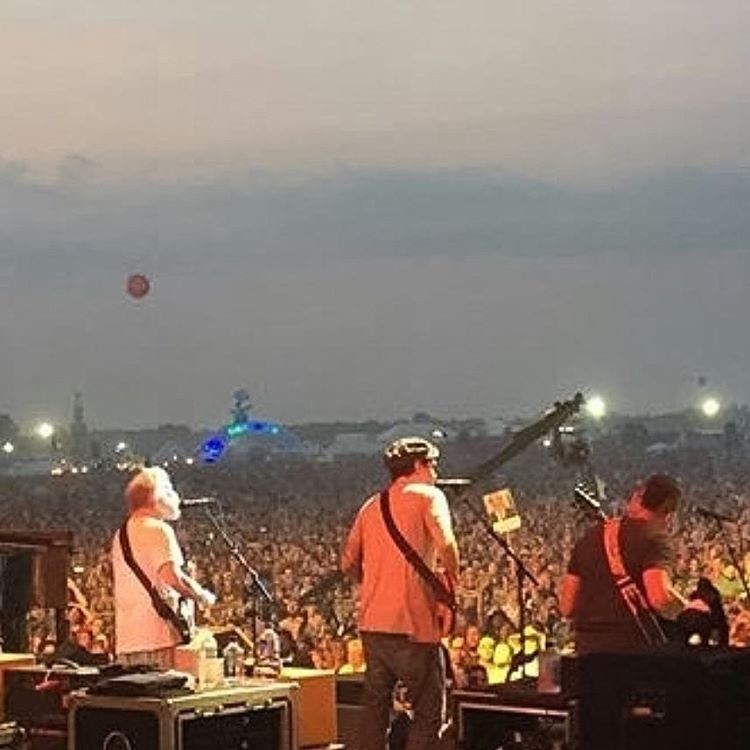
[570,481,608,525]
[177,559,195,642]
[436,566,455,638]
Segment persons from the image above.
[559,473,710,749]
[340,436,460,750]
[111,465,218,671]
[0,482,750,687]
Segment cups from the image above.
[200,657,224,686]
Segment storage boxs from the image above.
[0,653,338,750]
[537,653,585,695]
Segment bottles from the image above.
[254,623,282,680]
[537,639,562,694]
[222,636,245,688]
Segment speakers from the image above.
[248,666,337,746]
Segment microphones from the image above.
[435,478,472,486]
[697,506,738,524]
[180,499,216,508]
